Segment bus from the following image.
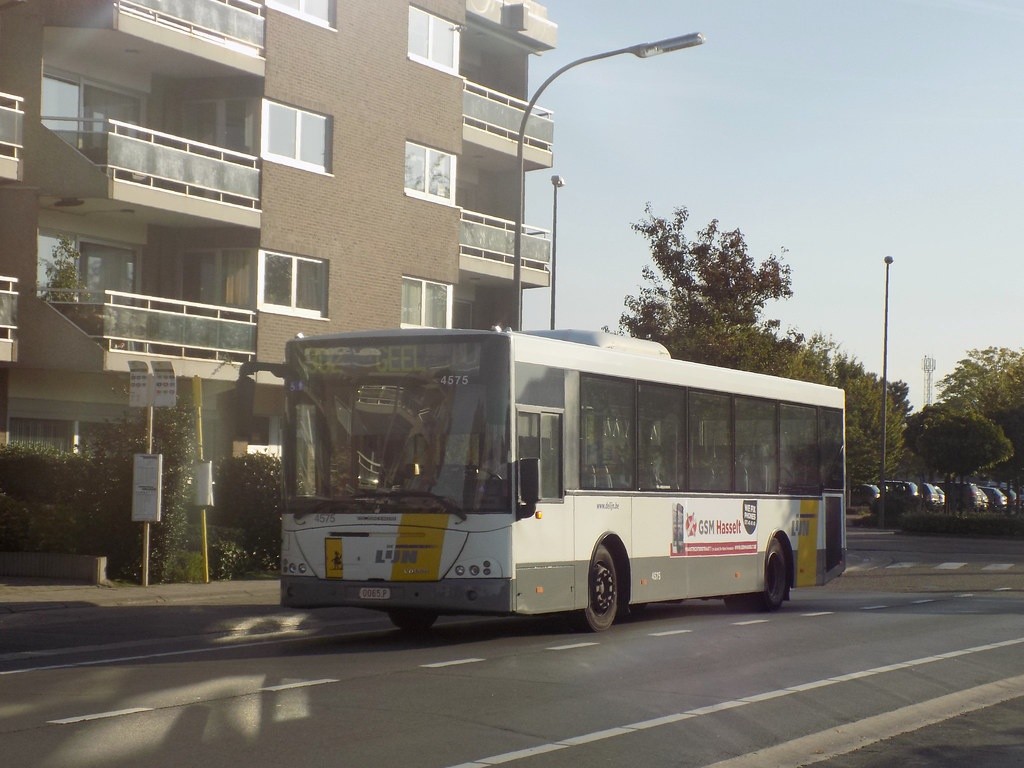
[275,325,848,634]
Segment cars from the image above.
[849,481,1024,515]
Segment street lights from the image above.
[513,29,707,332]
[549,174,562,329]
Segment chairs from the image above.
[580,452,823,489]
